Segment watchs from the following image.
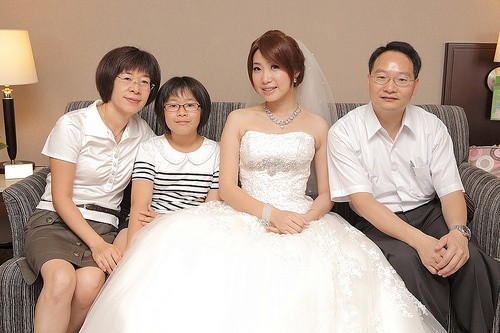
[449,224,471,241]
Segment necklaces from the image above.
[263,100,301,129]
[103,105,124,138]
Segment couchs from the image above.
[0,100,500,333]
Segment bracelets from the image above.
[261,203,273,226]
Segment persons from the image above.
[322,42,495,333]
[26,47,223,333]
[79,30,447,333]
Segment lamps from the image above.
[0,30,36,174]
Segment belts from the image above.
[36,199,121,219]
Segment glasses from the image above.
[369,70,416,87]
[116,75,156,92]
[164,102,202,111]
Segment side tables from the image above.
[0,165,47,247]
